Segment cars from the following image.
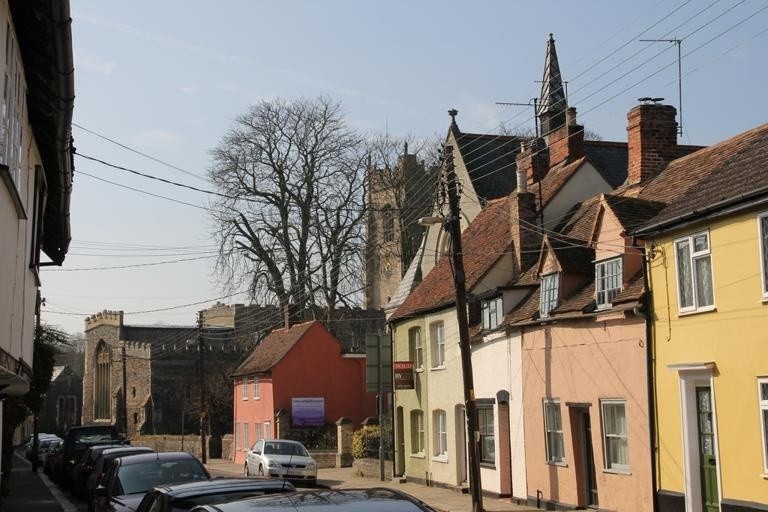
[95,452,212,512]
[136,477,298,512]
[244,438,318,480]
[189,487,434,512]
[28,424,159,501]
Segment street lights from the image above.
[418,214,490,512]
